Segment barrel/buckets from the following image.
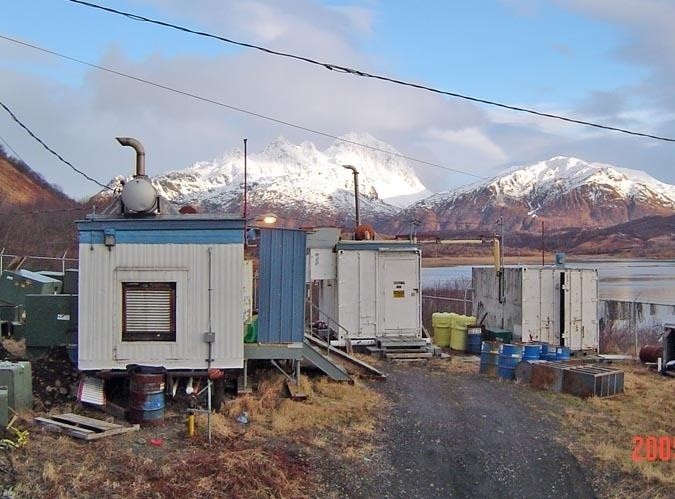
[128,372,165,427]
[432,312,571,381]
